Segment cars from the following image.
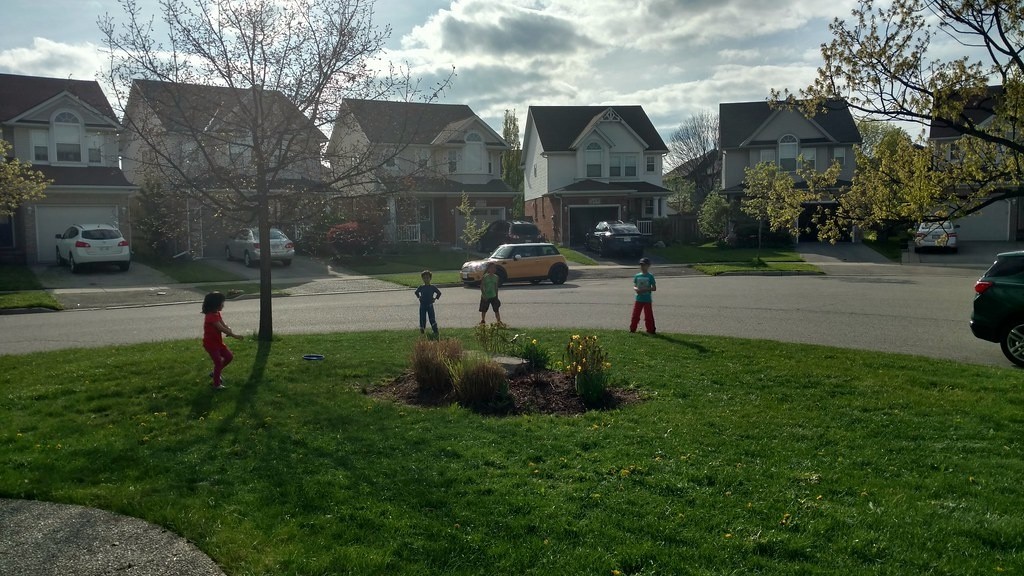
[969,251,1024,367]
[55,223,130,273]
[459,243,568,287]
[913,215,960,252]
[226,227,296,267]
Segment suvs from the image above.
[476,219,550,251]
[584,219,644,259]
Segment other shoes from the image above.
[481,320,486,324]
[497,322,504,326]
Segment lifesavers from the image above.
[302,354,326,360]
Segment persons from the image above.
[478,265,501,324]
[414,270,441,334]
[200,291,244,389]
[629,257,656,334]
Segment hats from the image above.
[639,257,651,265]
